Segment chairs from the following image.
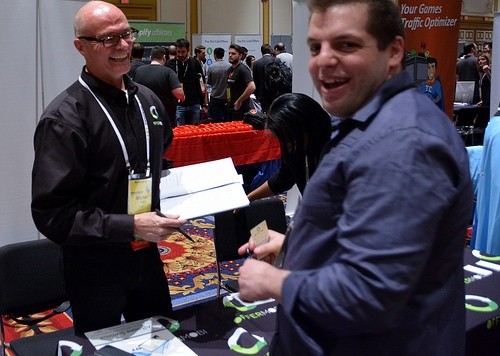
[213,199,290,296]
[0,237,79,338]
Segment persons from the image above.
[127,38,332,214]
[237,0,474,356]
[457,41,493,146]
[30,0,173,336]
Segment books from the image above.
[159,157,250,221]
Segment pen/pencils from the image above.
[246,248,252,258]
[154,208,196,244]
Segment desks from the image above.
[166,118,282,169]
[9,246,500,356]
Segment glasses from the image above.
[78,28,138,48]
